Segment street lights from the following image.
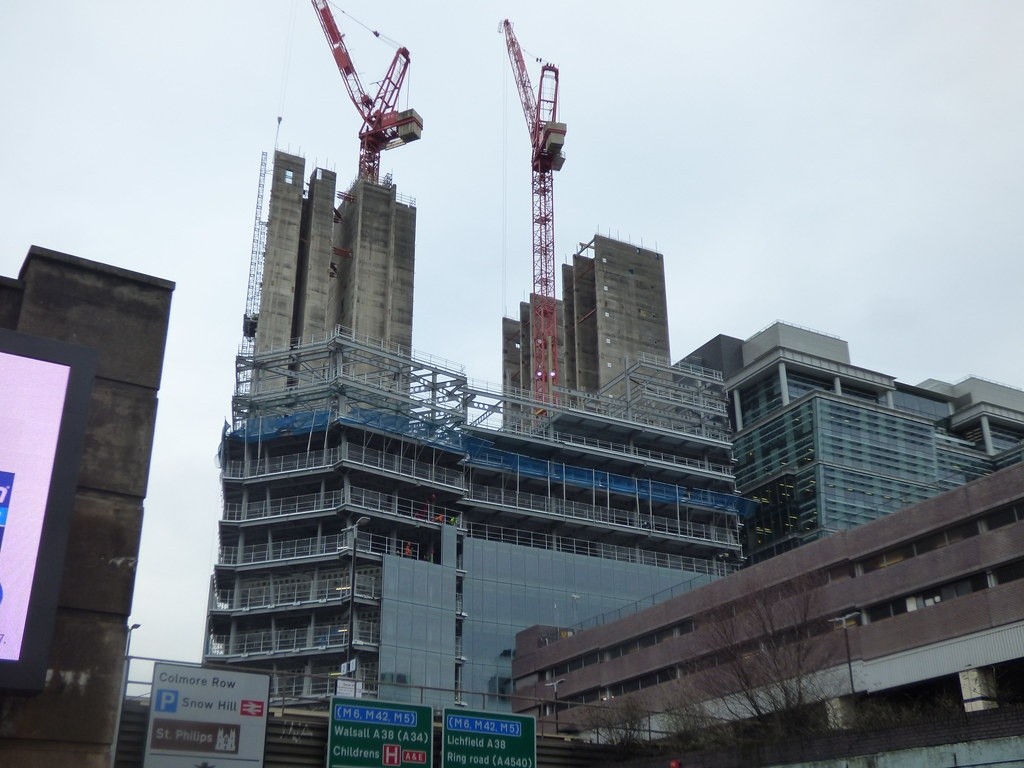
[108,623,145,768]
[339,514,372,679]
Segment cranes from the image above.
[494,17,571,419]
[230,149,270,418]
[308,0,424,187]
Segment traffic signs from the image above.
[441,706,542,768]
[326,696,435,768]
